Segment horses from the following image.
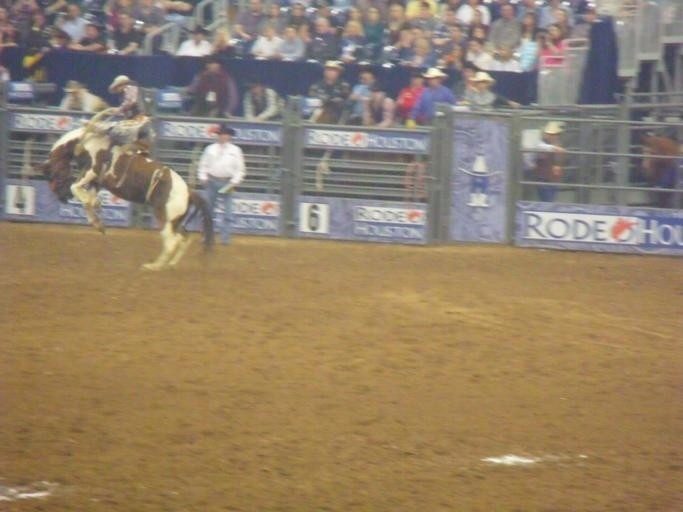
[638,133,683,178]
[34,127,213,271]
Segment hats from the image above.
[191,26,209,35]
[215,124,236,136]
[108,75,129,91]
[63,81,84,92]
[322,60,342,68]
[470,72,495,82]
[421,69,448,78]
[544,122,563,134]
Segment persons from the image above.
[1,0,681,217]
[195,122,246,249]
